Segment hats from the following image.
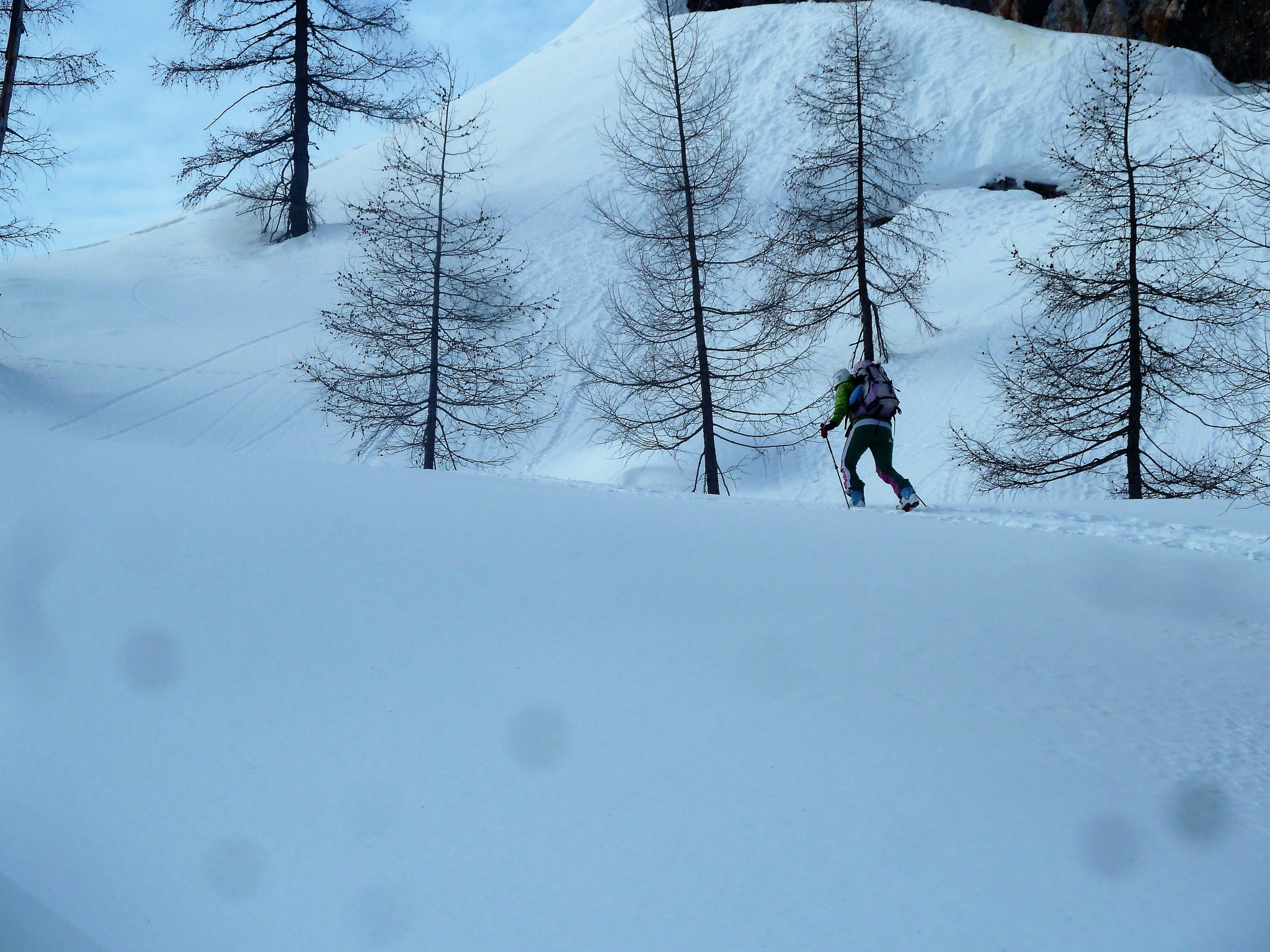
[832,368,850,392]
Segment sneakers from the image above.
[848,491,865,508]
[896,486,919,512]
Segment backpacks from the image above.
[849,359,901,421]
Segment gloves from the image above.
[821,421,837,439]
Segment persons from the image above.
[821,369,920,512]
